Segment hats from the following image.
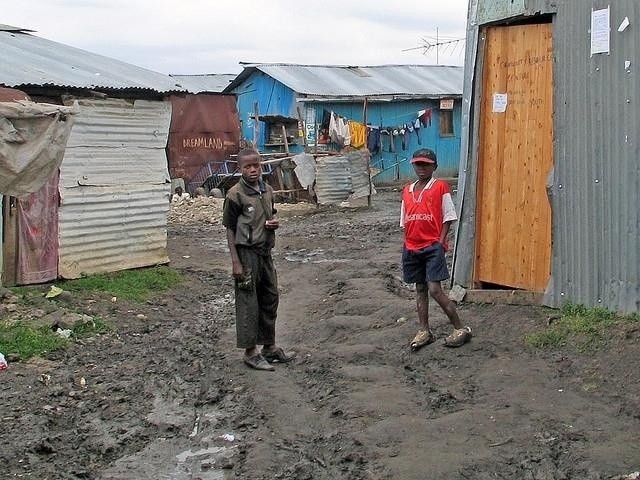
[408,147,438,165]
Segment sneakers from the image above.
[442,325,474,347]
[411,329,436,348]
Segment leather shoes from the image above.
[241,352,276,372]
[259,347,297,364]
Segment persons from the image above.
[400,148,472,352]
[221,147,297,372]
